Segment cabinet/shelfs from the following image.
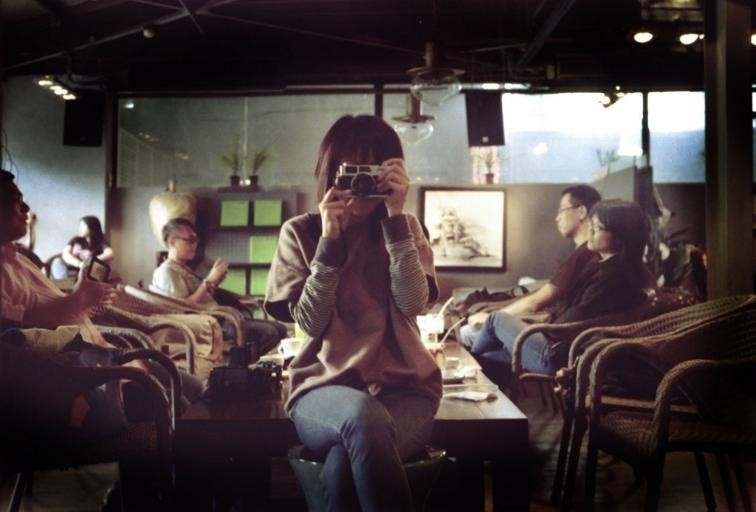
[190,187,305,338]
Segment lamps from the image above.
[390,92,433,146]
[404,40,462,105]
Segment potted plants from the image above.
[248,144,273,192]
[221,135,240,188]
[483,148,497,185]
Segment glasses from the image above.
[177,236,201,244]
[587,221,608,233]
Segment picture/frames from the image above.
[417,186,506,272]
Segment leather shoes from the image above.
[103,480,165,512]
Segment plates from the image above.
[441,374,468,383]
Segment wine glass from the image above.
[426,313,445,344]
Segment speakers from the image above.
[464,89,504,148]
[62,90,104,146]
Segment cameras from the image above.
[336,163,390,198]
[209,342,282,399]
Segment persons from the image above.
[150,217,288,365]
[470,199,653,388]
[263,113,443,511]
[458,184,603,350]
[0,166,211,511]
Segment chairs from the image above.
[44,253,110,294]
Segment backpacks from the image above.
[462,286,528,317]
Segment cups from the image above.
[444,357,466,376]
[277,337,305,355]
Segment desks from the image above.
[177,331,528,512]
